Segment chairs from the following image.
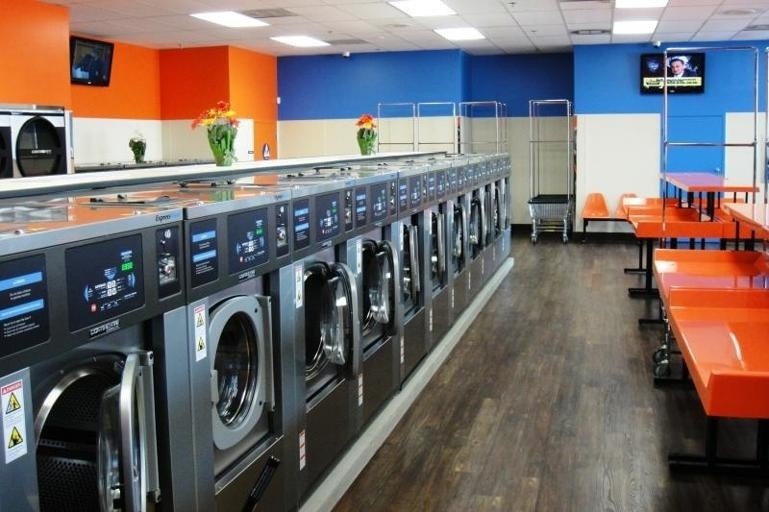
[581,192,745,247]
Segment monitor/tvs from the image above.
[640,50,706,95]
[69,34,115,87]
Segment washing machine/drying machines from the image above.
[1,192,198,511]
[183,170,361,506]
[73,181,299,511]
[292,150,513,511]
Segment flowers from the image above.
[191,100,241,166]
[355,113,377,155]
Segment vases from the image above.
[129,137,146,164]
[356,127,377,155]
[207,124,239,166]
